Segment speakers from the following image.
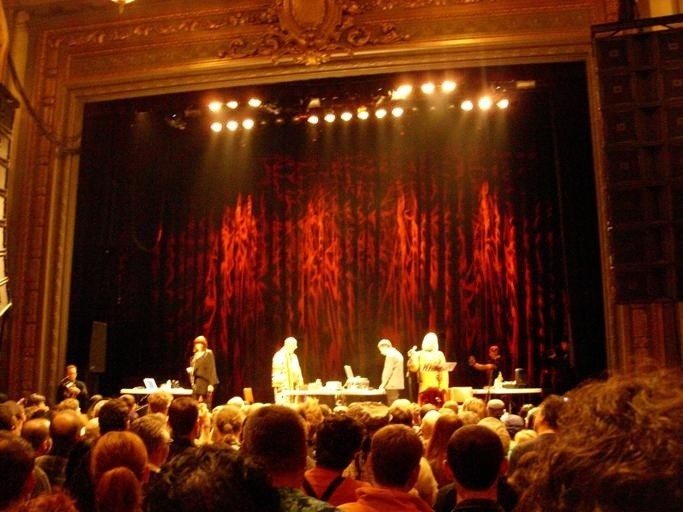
[342,391,388,404]
[289,390,339,406]
[475,389,545,406]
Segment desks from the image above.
[283,387,386,405]
[121,388,195,395]
[473,388,541,412]
